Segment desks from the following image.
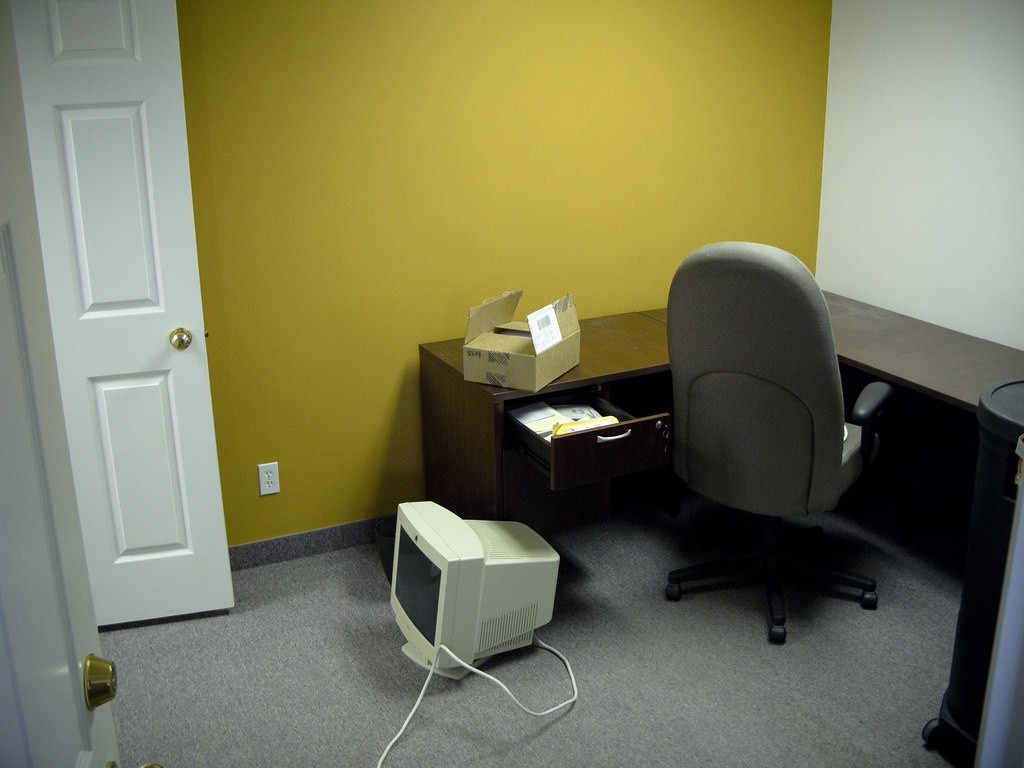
[419,286,1024,583]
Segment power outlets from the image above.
[257,461,281,497]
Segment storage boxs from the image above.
[462,288,581,392]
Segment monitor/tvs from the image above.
[390,501,560,680]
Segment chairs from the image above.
[665,239,876,645]
